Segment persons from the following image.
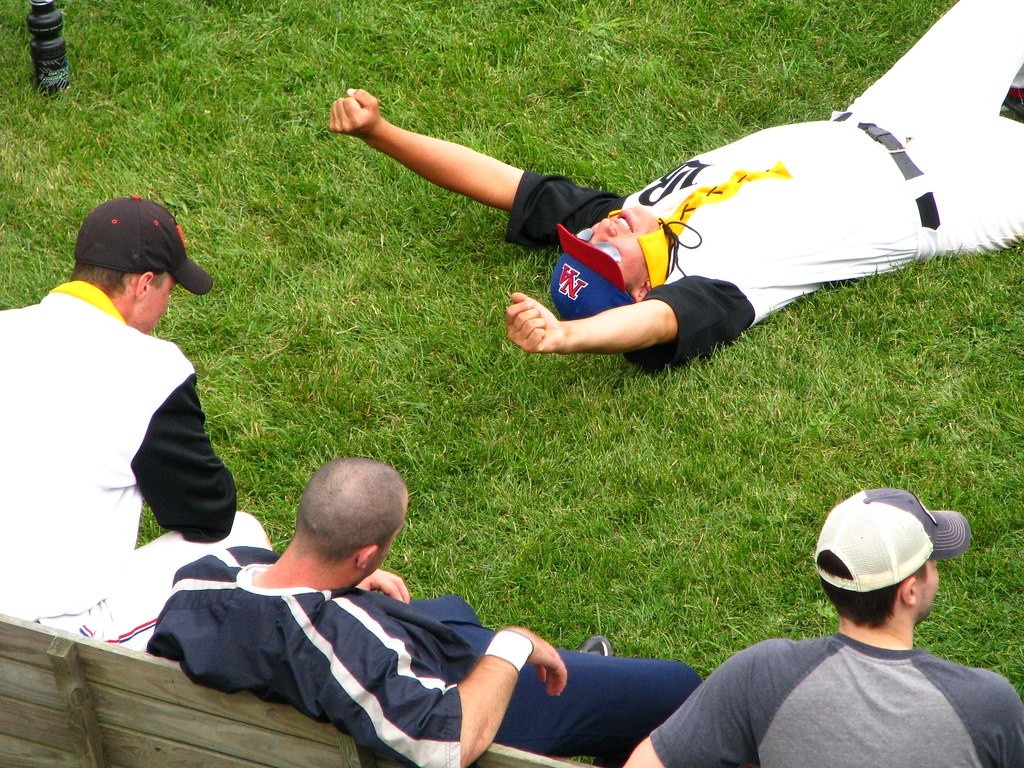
[0,194,272,653]
[147,457,702,768]
[329,0,1024,371]
[624,487,1024,768]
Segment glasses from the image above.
[574,227,622,263]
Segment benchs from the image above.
[1,612,594,768]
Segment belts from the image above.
[833,112,940,230]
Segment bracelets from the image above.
[484,629,534,672]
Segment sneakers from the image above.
[577,635,615,656]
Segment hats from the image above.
[74,195,213,295]
[814,488,971,592]
[550,224,635,319]
